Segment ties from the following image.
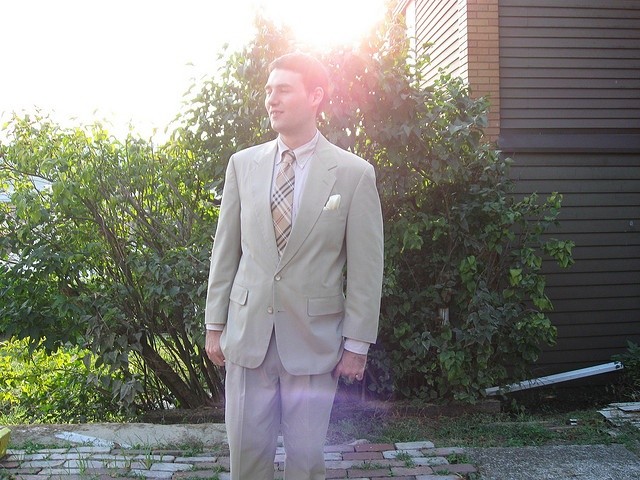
[270,151,295,258]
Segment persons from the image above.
[204,52,385,479]
